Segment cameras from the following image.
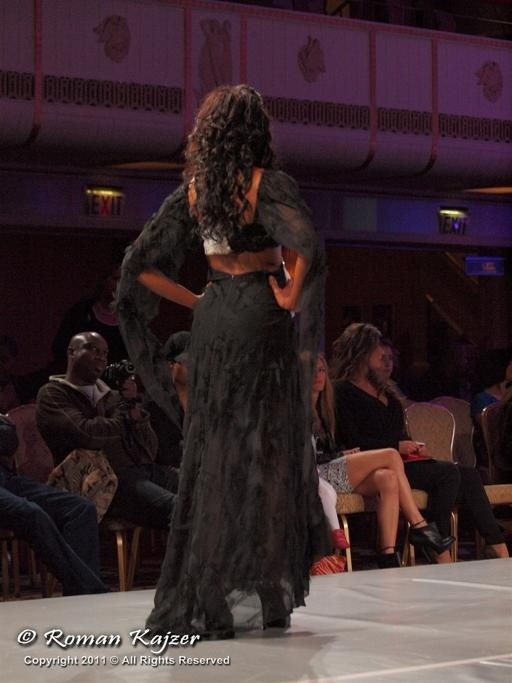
[104,360,137,388]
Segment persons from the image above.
[317,477,351,551]
[469,348,512,425]
[327,324,510,563]
[297,351,456,567]
[114,83,324,642]
[379,340,394,382]
[34,331,180,531]
[142,332,190,467]
[0,420,106,595]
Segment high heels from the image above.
[409,519,455,563]
[375,547,399,568]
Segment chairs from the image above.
[403,401,510,562]
[479,399,512,484]
[325,474,429,570]
[4,404,153,592]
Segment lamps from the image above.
[437,207,466,217]
[84,167,132,200]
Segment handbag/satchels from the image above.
[45,449,117,524]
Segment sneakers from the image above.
[328,530,351,549]
